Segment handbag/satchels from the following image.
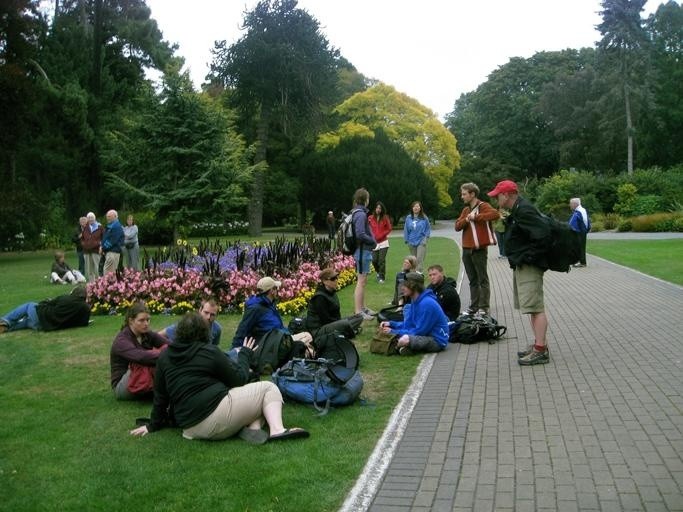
[124,242,138,250]
[467,201,499,250]
[369,332,401,356]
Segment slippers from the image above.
[269,427,310,442]
[237,425,268,447]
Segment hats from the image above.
[317,268,338,281]
[255,276,282,295]
[485,181,518,198]
[398,272,424,291]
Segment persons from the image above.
[393,254,423,305]
[47,250,78,286]
[485,179,551,367]
[336,211,348,222]
[378,269,453,356]
[71,208,141,281]
[0,284,91,335]
[366,201,392,285]
[401,199,432,272]
[110,270,341,398]
[566,197,591,268]
[425,264,462,321]
[492,204,509,260]
[453,182,501,319]
[325,210,336,240]
[348,188,378,322]
[130,313,310,444]
[487,221,496,246]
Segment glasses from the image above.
[325,276,338,281]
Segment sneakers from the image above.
[352,312,373,320]
[361,306,377,317]
[517,339,549,358]
[460,306,476,315]
[516,347,549,365]
[472,307,490,317]
[71,281,79,286]
[0,320,8,333]
[58,280,68,286]
[375,272,385,285]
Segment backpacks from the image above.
[270,355,366,410]
[512,200,582,274]
[334,208,368,256]
[447,313,508,344]
[376,304,409,324]
